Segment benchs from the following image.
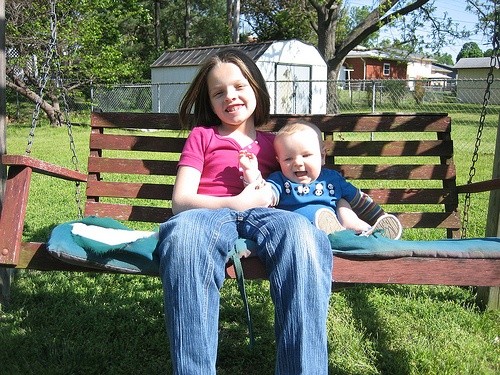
[1,112,500,286]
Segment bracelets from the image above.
[241,171,261,186]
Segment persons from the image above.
[235,116,404,240]
[157,47,336,375]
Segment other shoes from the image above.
[313,208,346,235]
[359,214,402,240]
